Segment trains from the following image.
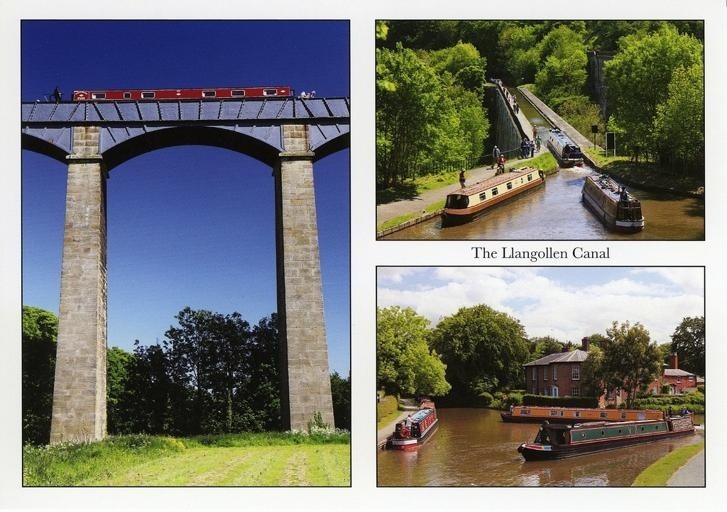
[69,83,295,101]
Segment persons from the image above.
[491,145,500,168]
[405,414,414,438]
[680,405,689,416]
[531,125,537,138]
[668,407,672,417]
[495,154,505,173]
[521,137,534,159]
[459,168,466,190]
[534,135,541,151]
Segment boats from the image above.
[545,124,584,170]
[516,412,696,462]
[497,404,664,424]
[440,164,545,220]
[579,172,645,234]
[389,399,438,451]
